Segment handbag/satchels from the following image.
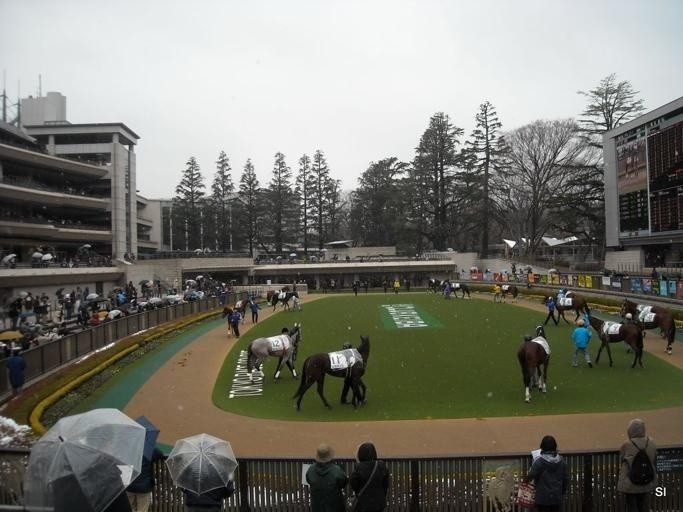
[516,482,537,508]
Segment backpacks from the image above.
[623,437,653,486]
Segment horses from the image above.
[247,322,301,384]
[221,284,299,336]
[500,284,518,303]
[588,316,644,368]
[544,288,591,325]
[293,335,370,411]
[517,324,552,403]
[617,297,675,356]
[426,278,471,299]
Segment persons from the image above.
[523,335,538,389]
[570,319,594,367]
[522,436,569,511]
[0,242,233,357]
[105,492,132,512]
[616,419,657,512]
[230,307,243,338]
[249,298,262,325]
[7,347,28,395]
[294,295,302,312]
[273,327,297,378]
[340,342,358,399]
[179,443,233,511]
[461,261,561,326]
[259,251,454,300]
[125,447,163,512]
[619,312,638,354]
[345,443,390,512]
[306,445,349,512]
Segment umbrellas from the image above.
[21,409,146,511]
[136,416,160,468]
[168,435,238,495]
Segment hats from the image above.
[313,444,336,463]
